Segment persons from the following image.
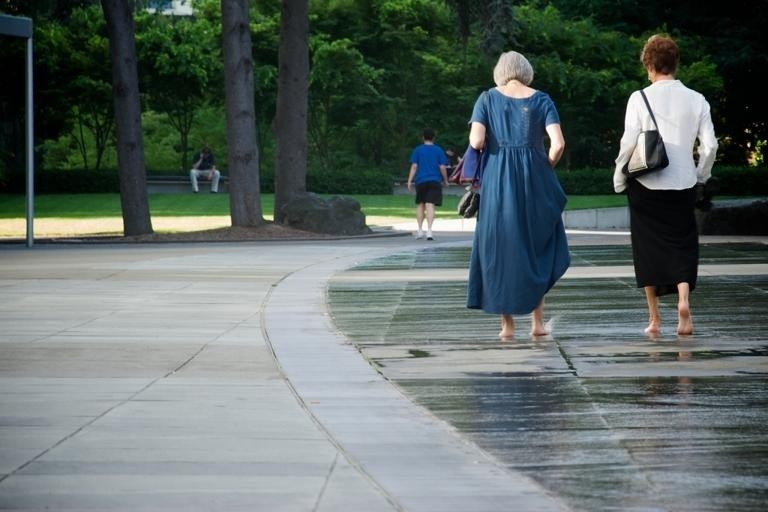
[611,33,720,335]
[189,146,220,194]
[406,127,449,240]
[461,51,573,341]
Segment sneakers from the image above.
[416,232,434,240]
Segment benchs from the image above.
[393,178,477,196]
[144,175,231,192]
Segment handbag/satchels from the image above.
[457,152,484,218]
[621,89,669,179]
